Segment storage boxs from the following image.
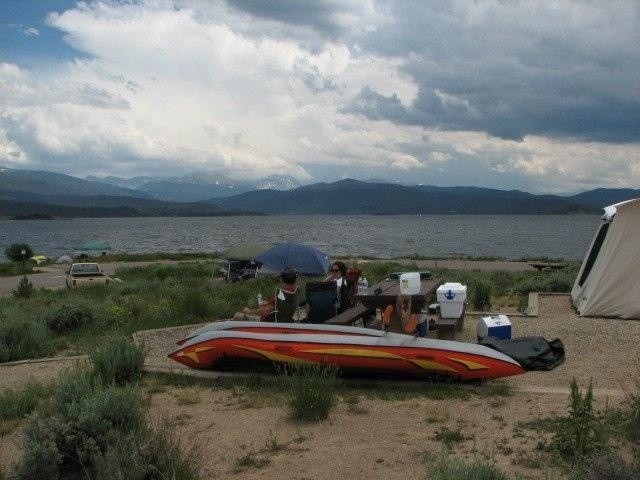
[436,282,467,319]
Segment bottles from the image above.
[357,277,362,296]
[256,290,262,304]
[362,278,368,295]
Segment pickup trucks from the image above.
[66,263,107,289]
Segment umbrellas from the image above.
[253,242,332,283]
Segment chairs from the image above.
[243,278,353,323]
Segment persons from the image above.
[300,262,347,318]
[233,266,298,320]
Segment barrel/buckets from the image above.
[400,271,421,295]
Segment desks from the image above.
[353,277,443,334]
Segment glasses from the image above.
[331,268,339,273]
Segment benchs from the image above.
[324,303,466,340]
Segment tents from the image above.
[571,198,640,319]
[55,254,73,264]
